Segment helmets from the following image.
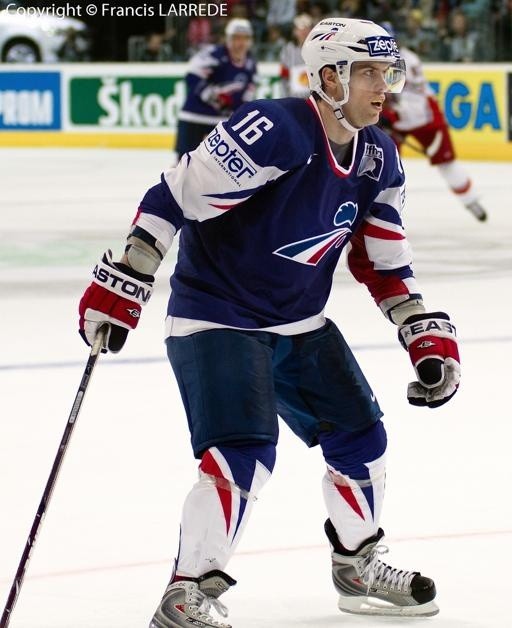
[293,12,313,29]
[299,15,404,94]
[226,17,252,38]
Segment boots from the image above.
[320,516,440,607]
[148,552,238,628]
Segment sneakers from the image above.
[464,200,488,222]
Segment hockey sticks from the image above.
[385,129,442,157]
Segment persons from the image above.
[376,48,491,225]
[175,18,260,163]
[53,1,512,97]
[73,13,465,628]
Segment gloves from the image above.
[77,244,161,356]
[200,85,232,113]
[392,309,465,413]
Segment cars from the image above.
[0,12,89,62]
